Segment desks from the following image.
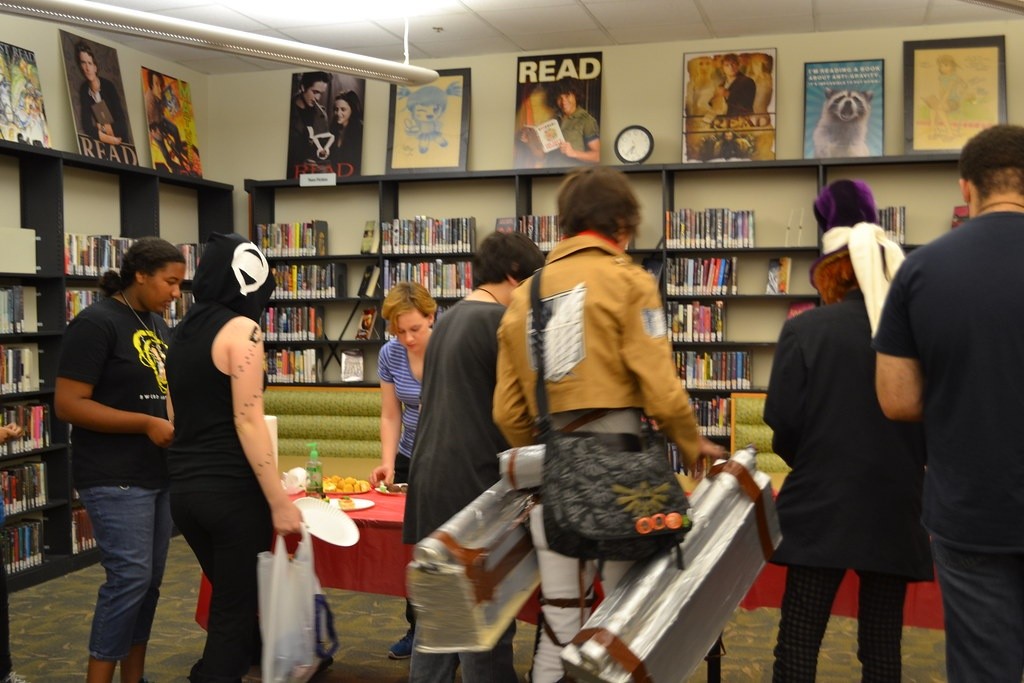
[194,480,722,683]
[704,487,945,683]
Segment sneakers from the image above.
[389,629,414,658]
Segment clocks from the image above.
[614,125,654,164]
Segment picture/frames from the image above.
[802,59,885,159]
[385,67,472,176]
[903,35,1008,155]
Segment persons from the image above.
[491,166,727,681]
[145,71,188,173]
[54,237,187,683]
[164,231,304,683]
[0,422,29,683]
[699,53,756,159]
[873,125,1024,683]
[288,71,363,179]
[519,76,600,164]
[75,41,129,164]
[367,232,546,683]
[763,178,925,683]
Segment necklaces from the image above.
[476,287,499,303]
[120,291,156,340]
[978,201,1024,215]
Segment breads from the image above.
[386,484,408,493]
[322,477,369,493]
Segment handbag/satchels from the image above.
[257,521,340,683]
[540,432,694,571]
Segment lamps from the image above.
[0,0,441,88]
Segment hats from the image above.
[958,125,1024,181]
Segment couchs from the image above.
[729,393,796,491]
[263,385,407,482]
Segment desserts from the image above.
[338,496,355,510]
[320,494,330,504]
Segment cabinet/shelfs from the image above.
[0,138,961,592]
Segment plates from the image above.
[329,498,375,511]
[374,483,408,495]
[292,496,360,547]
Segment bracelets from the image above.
[101,122,106,133]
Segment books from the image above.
[523,118,566,154]
[356,215,477,341]
[256,220,347,382]
[0,231,198,575]
[666,207,755,480]
[495,217,516,232]
[765,257,791,294]
[878,206,905,245]
[519,214,561,243]
[307,125,332,144]
[91,100,114,125]
